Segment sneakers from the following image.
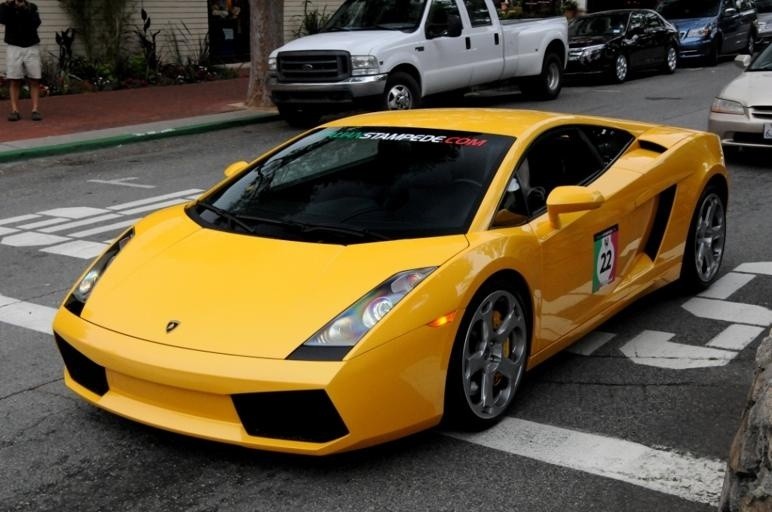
[8,112,22,121]
[32,111,42,121]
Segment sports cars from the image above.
[53,108,729,456]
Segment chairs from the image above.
[597,17,612,34]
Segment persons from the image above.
[1,0,44,121]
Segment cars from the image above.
[562,0,772,149]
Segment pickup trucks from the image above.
[268,1,569,130]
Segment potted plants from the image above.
[561,0,578,18]
